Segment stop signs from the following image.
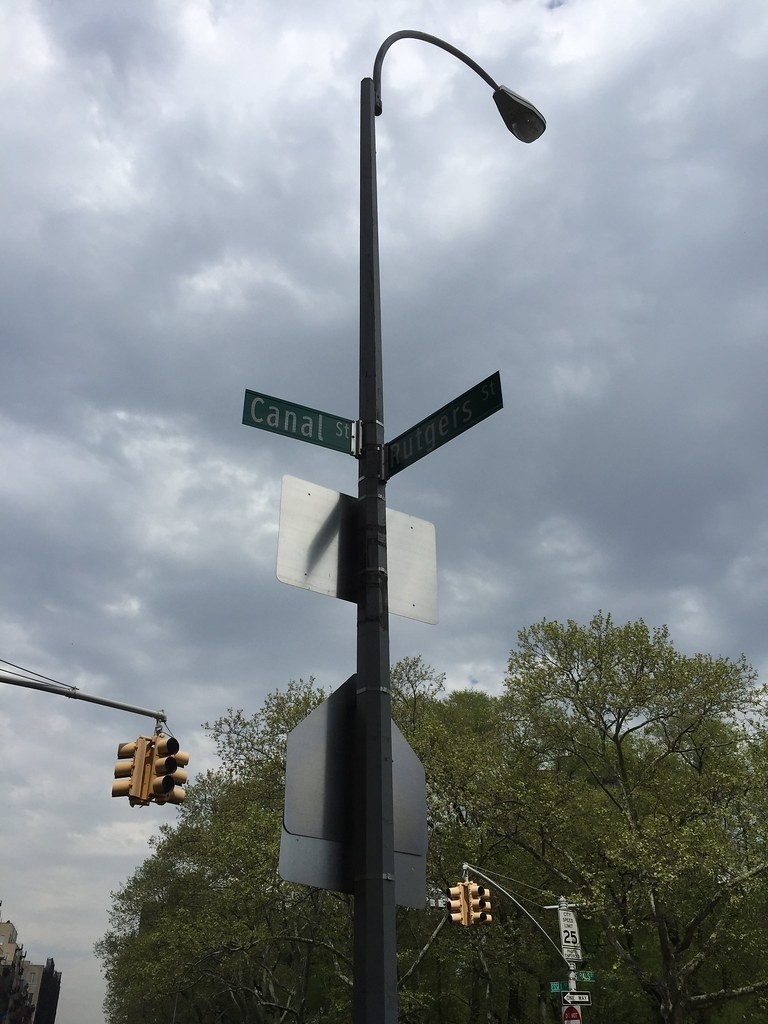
[562,1006,582,1024]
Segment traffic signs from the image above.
[576,971,596,982]
[550,981,569,993]
[560,991,592,1006]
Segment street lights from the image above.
[352,28,548,1024]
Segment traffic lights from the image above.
[445,884,467,926]
[148,734,191,805]
[112,739,149,799]
[468,881,493,927]
